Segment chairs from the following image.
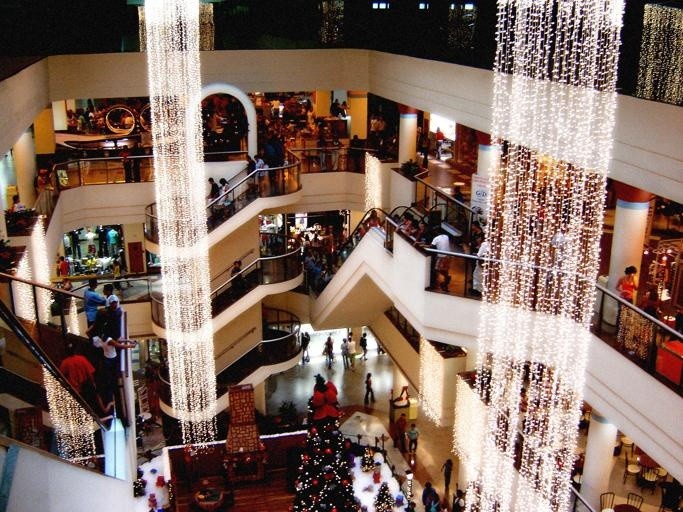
[262,92,458,170]
[469,362,683,511]
